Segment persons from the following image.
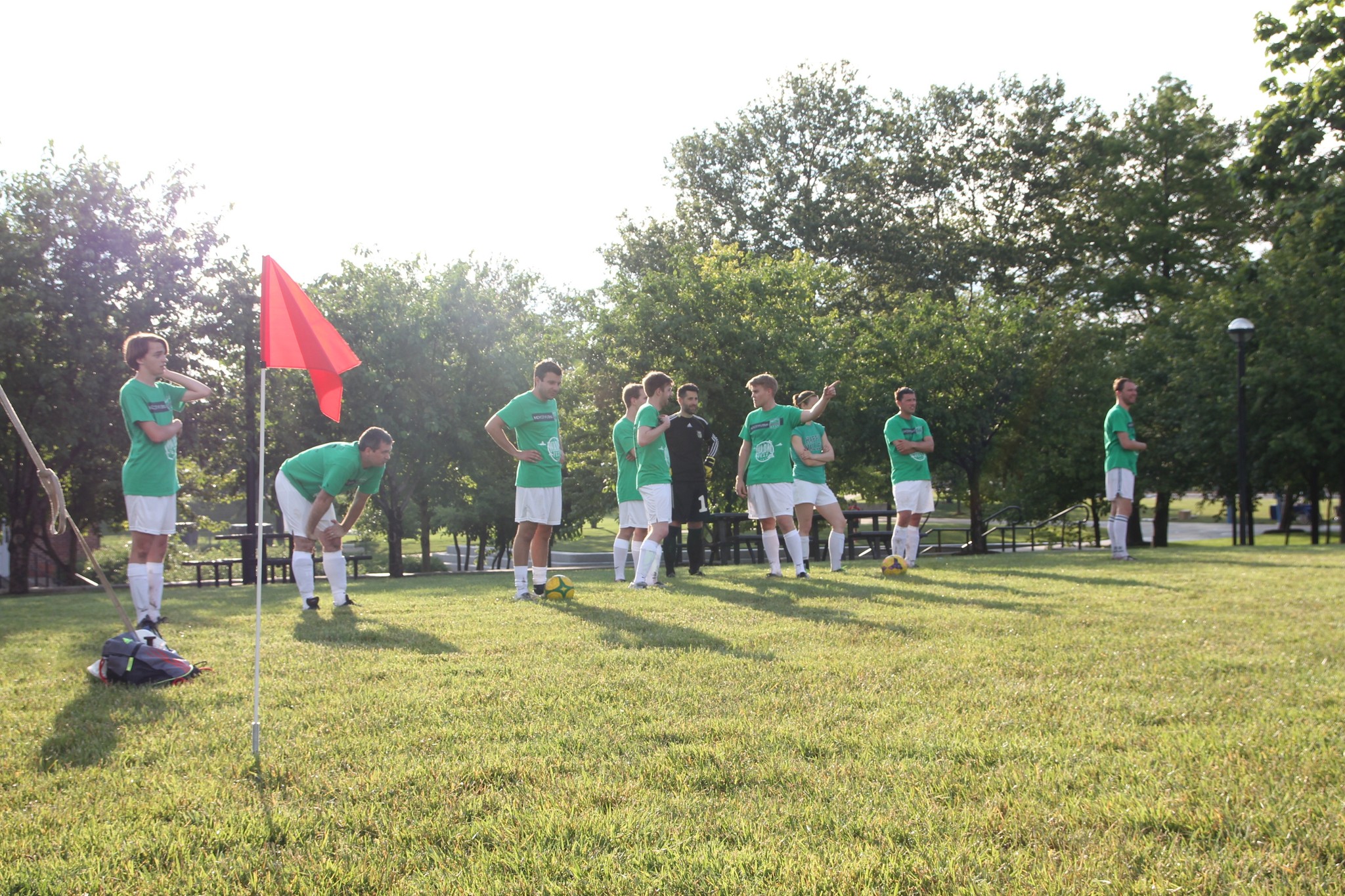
[790,390,846,573]
[1103,377,1147,563]
[849,500,861,544]
[608,370,719,588]
[735,370,839,580]
[274,427,396,609]
[119,333,212,635]
[484,358,566,601]
[884,386,934,568]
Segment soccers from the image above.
[543,574,575,601]
[880,554,909,577]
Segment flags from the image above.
[261,256,362,425]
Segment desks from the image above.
[792,509,897,562]
[213,533,292,585]
[703,513,754,564]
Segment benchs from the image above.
[845,530,929,560]
[180,556,243,589]
[312,555,374,579]
[732,533,793,565]
[215,556,292,584]
[660,542,723,566]
[817,538,851,562]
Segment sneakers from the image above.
[333,595,361,605]
[533,590,544,598]
[653,582,666,587]
[517,592,533,600]
[303,597,321,609]
[628,581,647,589]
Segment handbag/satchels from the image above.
[98,633,195,683]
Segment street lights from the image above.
[1225,317,1258,546]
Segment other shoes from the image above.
[805,569,810,573]
[1119,556,1135,561]
[691,570,705,575]
[833,568,847,574]
[797,572,809,578]
[138,614,152,627]
[668,573,675,577]
[614,579,626,582]
[766,573,784,578]
[913,564,918,568]
[156,615,167,624]
